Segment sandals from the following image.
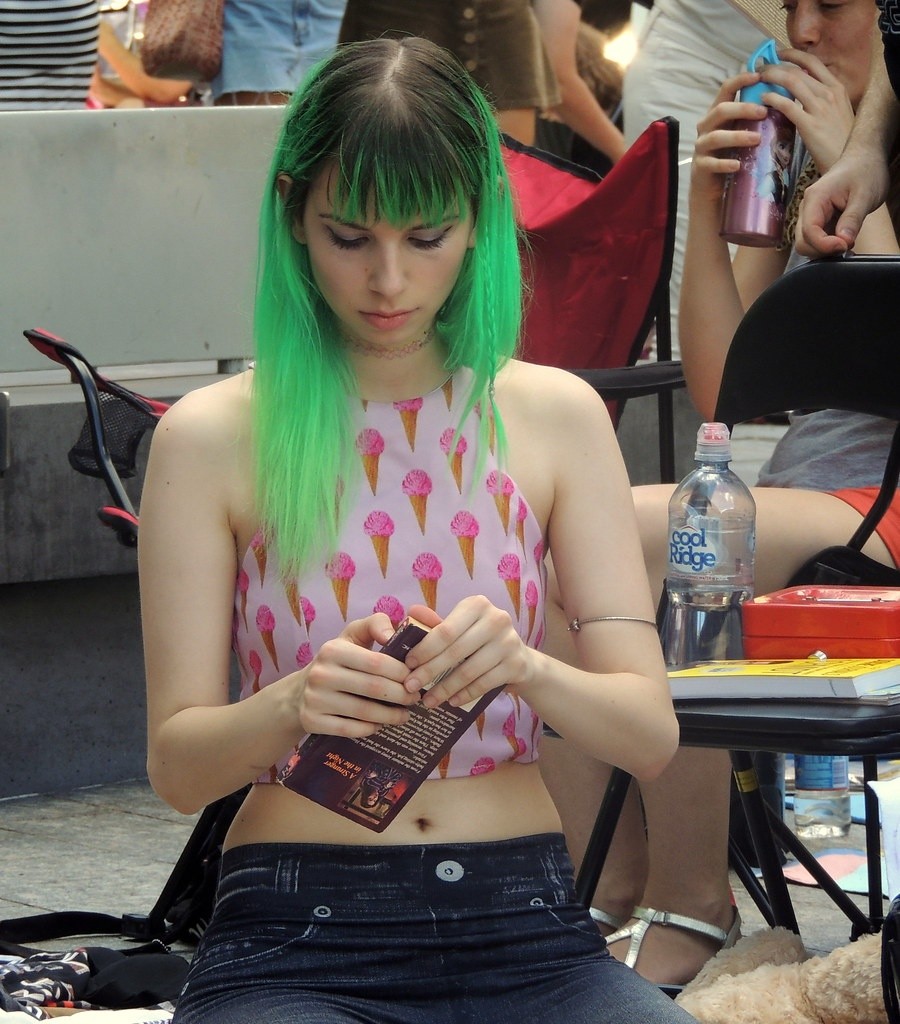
[604,905,742,995]
[590,909,622,932]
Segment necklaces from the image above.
[334,327,434,363]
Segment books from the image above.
[276,617,509,834]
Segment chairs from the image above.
[22,115,900,1024]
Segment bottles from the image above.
[665,422,756,666]
[794,753,851,838]
[719,38,797,247]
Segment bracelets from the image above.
[562,613,661,634]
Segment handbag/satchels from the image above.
[150,782,252,947]
[141,0,225,82]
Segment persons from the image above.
[0,0,900,1024]
